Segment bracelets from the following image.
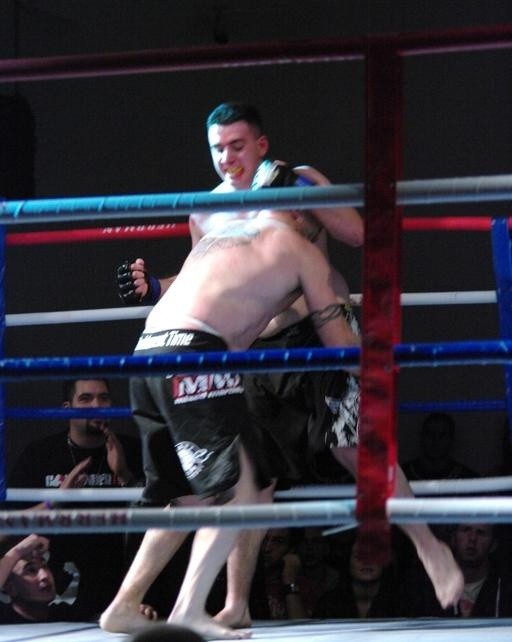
[44,501,55,510]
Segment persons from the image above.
[9,378,148,622]
[0,532,82,623]
[99,207,360,640]
[113,100,465,624]
[129,625,207,642]
[118,485,226,617]
[249,414,511,618]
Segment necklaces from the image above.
[68,437,105,477]
